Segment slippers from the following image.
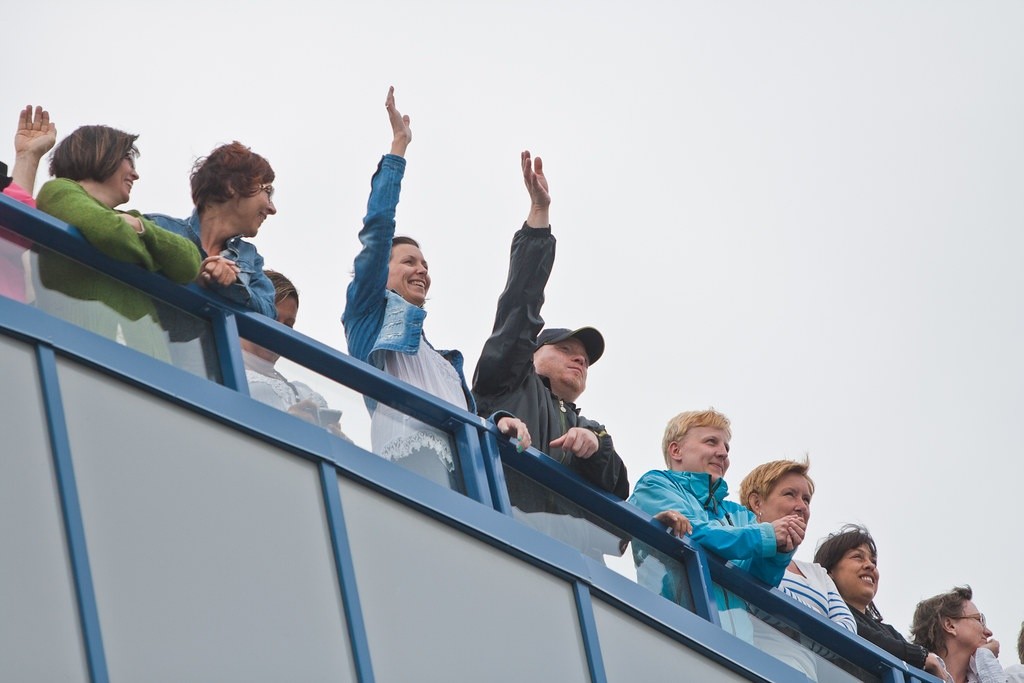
[249,185,272,202]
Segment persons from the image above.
[342,87,530,509]
[0,103,354,445]
[468,150,632,572]
[620,406,1023,683]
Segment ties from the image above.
[533,327,605,366]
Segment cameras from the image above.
[306,407,341,428]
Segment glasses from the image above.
[950,612,986,626]
[123,153,133,169]
